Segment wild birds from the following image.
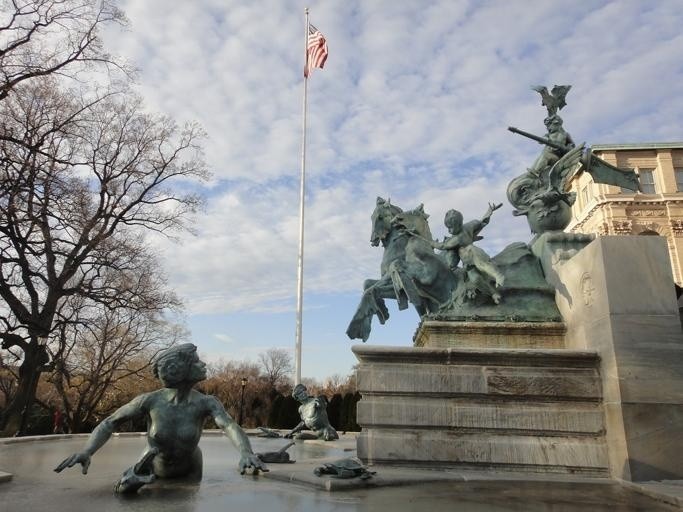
[534,141,587,208]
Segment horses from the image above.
[345,195,463,343]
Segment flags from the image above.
[304,22,330,77]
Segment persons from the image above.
[49,342,270,502]
[430,201,508,305]
[526,114,575,178]
[284,381,340,443]
